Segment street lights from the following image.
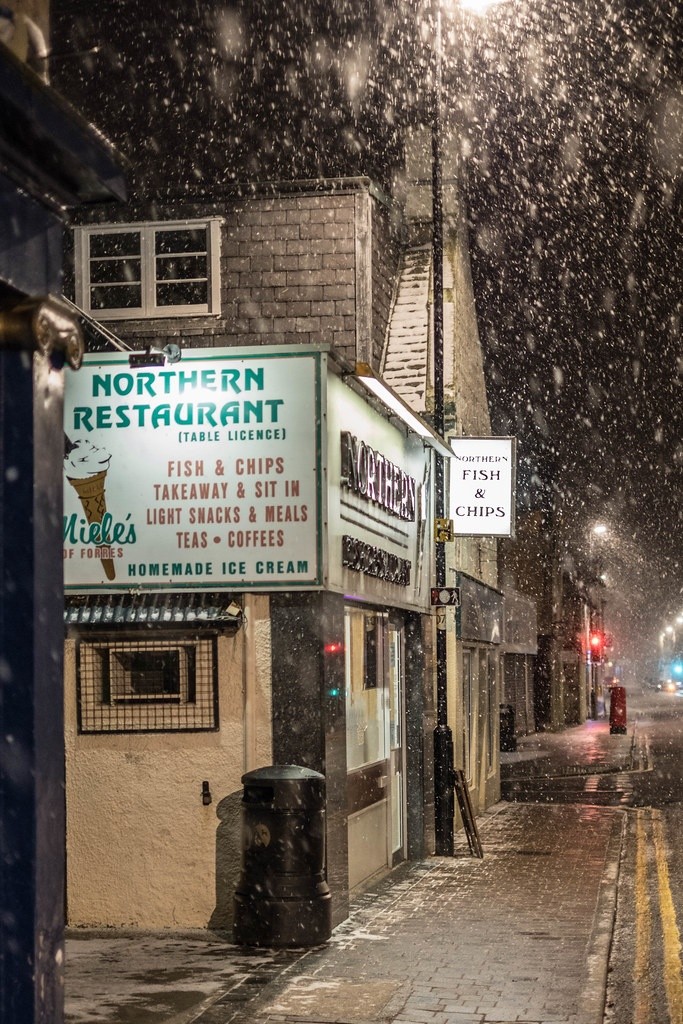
[430,1,506,855]
[666,612,683,648]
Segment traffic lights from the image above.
[590,630,600,661]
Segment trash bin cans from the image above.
[498,703,519,753]
[230,760,331,946]
